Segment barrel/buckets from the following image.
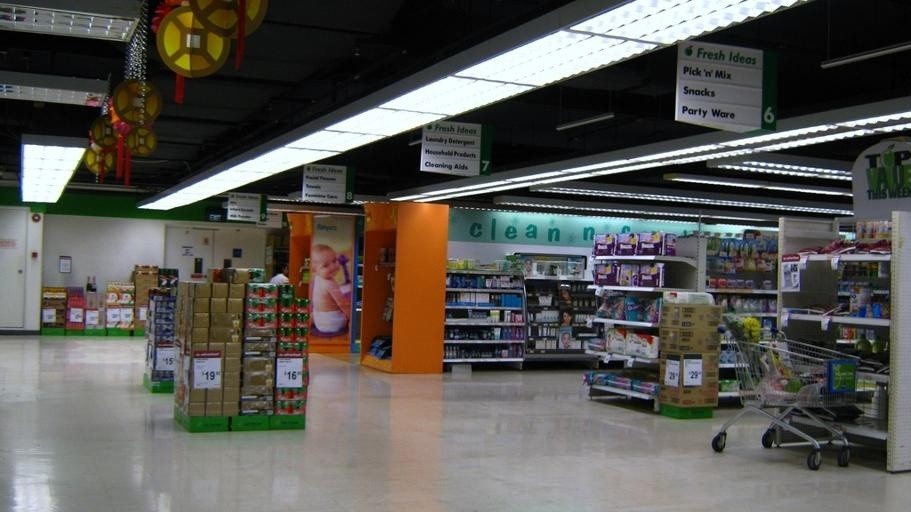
[854,333,872,354]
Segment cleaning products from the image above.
[705,235,778,258]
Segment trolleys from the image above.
[711,319,861,472]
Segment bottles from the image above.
[42,286,67,308]
[85,275,136,292]
[444,345,492,360]
[856,333,884,354]
[65,286,85,329]
[849,281,872,317]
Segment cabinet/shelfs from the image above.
[699,235,779,404]
[443,269,527,364]
[522,275,606,368]
[359,198,451,376]
[509,252,588,276]
[770,213,911,474]
[283,209,359,354]
[265,245,289,282]
[584,229,707,416]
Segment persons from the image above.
[311,243,353,335]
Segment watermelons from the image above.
[786,377,802,392]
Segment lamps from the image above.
[17,133,91,207]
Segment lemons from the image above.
[881,150,895,167]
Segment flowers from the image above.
[725,312,762,355]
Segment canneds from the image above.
[763,280,772,290]
[709,278,754,289]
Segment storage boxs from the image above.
[659,300,724,327]
[660,381,723,411]
[592,232,678,356]
[660,322,723,352]
[658,349,721,386]
